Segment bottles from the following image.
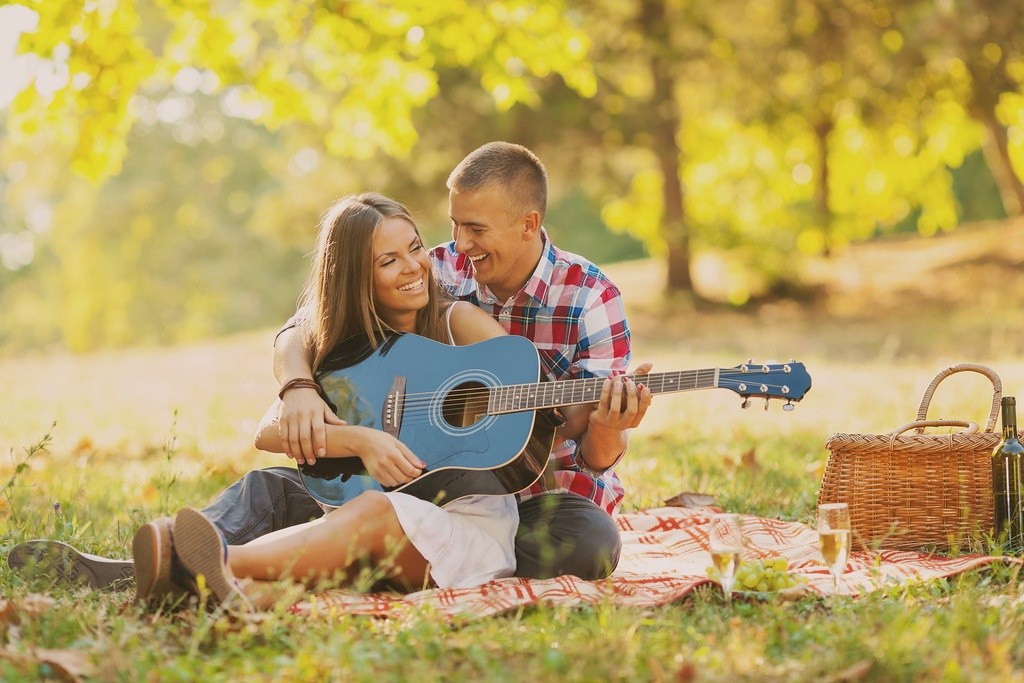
[992,397,1024,557]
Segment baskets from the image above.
[816,363,1024,551]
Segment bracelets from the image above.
[278,378,323,400]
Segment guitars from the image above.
[292,326,816,516]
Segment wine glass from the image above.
[707,515,741,608]
[817,502,852,594]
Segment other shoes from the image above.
[132,521,182,609]
[174,507,255,620]
[7,539,136,594]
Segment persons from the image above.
[8,140,653,597]
[132,191,653,609]
